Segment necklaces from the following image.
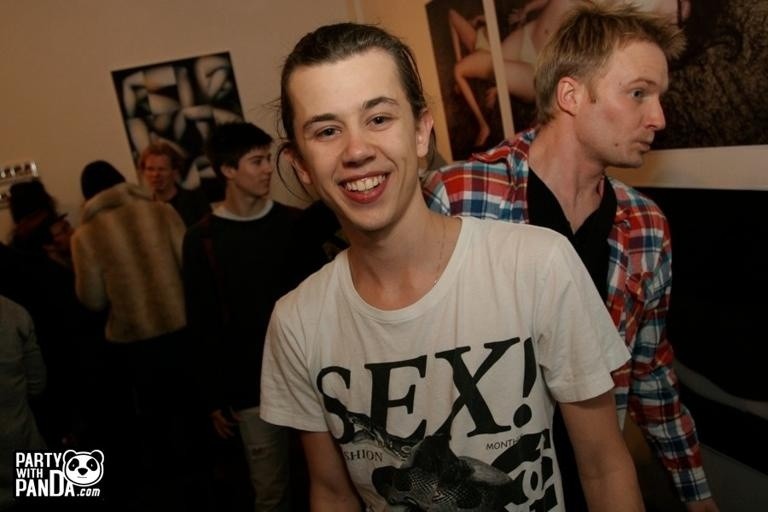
[432,214,447,286]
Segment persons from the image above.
[1,175,110,457]
[0,292,54,511]
[136,138,212,232]
[180,121,336,511]
[413,4,723,510]
[69,160,197,437]
[444,1,741,148]
[257,20,655,510]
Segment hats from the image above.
[79,159,126,202]
